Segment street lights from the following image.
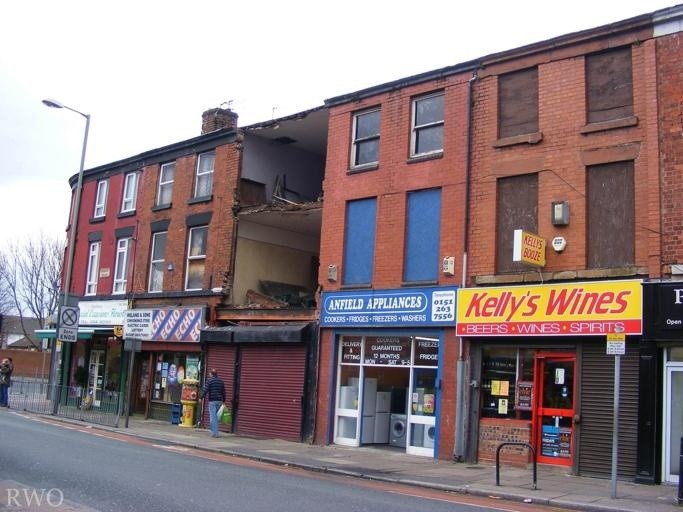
[41,98,93,416]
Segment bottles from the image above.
[480,359,515,389]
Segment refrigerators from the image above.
[337,376,390,445]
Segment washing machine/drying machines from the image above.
[423,424,435,449]
[389,414,412,448]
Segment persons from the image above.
[0,357,14,407]
[199,368,226,438]
